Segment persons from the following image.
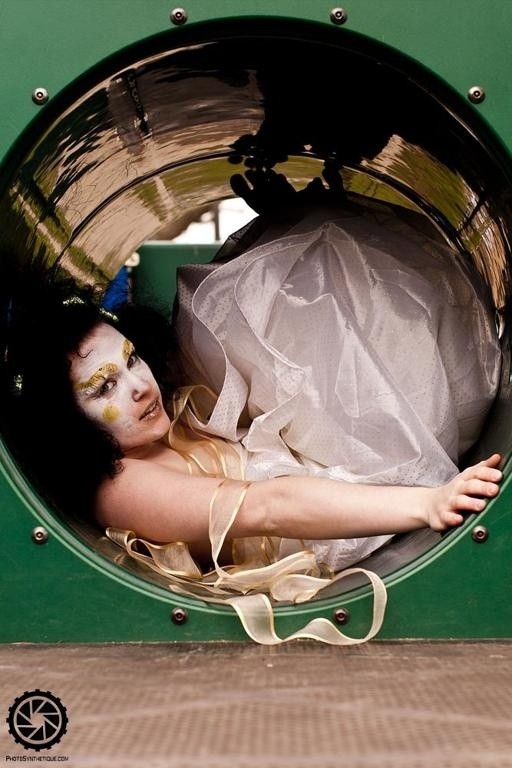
[1,170,503,649]
